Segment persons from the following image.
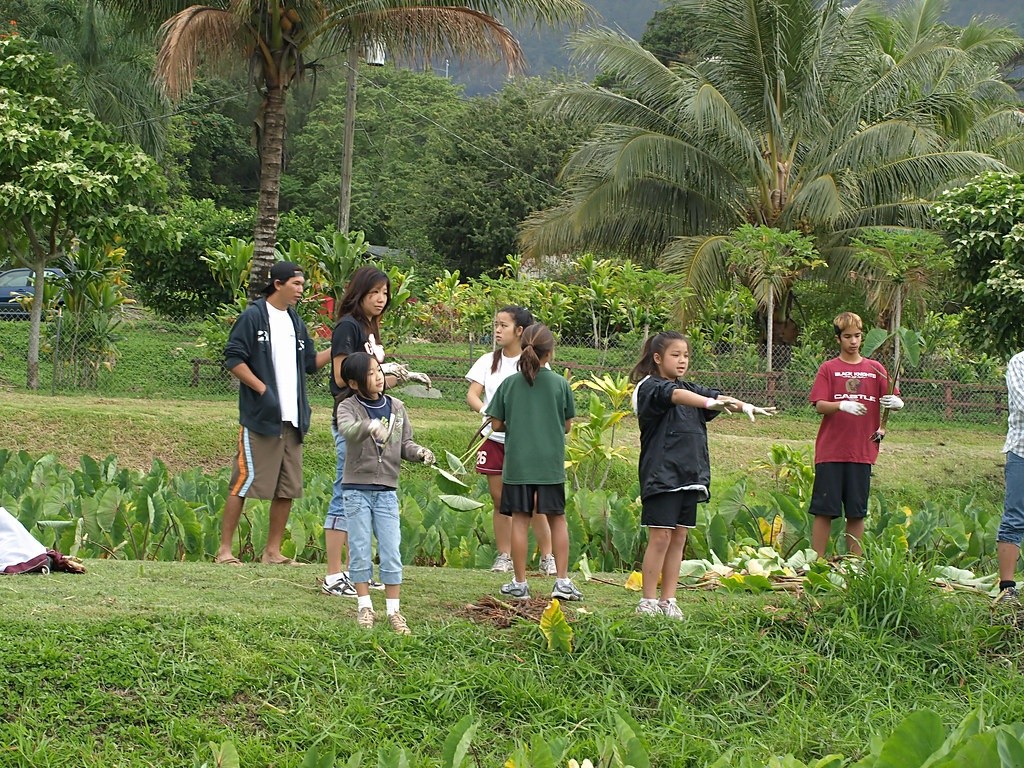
[321,267,432,597]
[630,329,777,620]
[334,352,436,637]
[465,307,557,577]
[810,312,904,561]
[997,351,1024,603]
[216,260,331,564]
[485,324,584,601]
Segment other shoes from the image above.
[999,587,1019,606]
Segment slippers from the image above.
[262,558,306,566]
[214,557,242,567]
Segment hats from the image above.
[262,261,305,294]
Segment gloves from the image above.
[839,400,867,416]
[741,403,777,423]
[416,447,436,464]
[367,420,389,444]
[379,361,409,380]
[879,394,905,410]
[705,398,738,415]
[397,371,432,391]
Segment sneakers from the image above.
[635,598,664,618]
[539,554,557,575]
[659,597,684,621]
[551,574,584,601]
[366,578,385,591]
[358,605,377,629]
[385,610,411,635]
[322,570,358,597]
[499,577,532,600]
[490,552,513,573]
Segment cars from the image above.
[0,267,73,321]
